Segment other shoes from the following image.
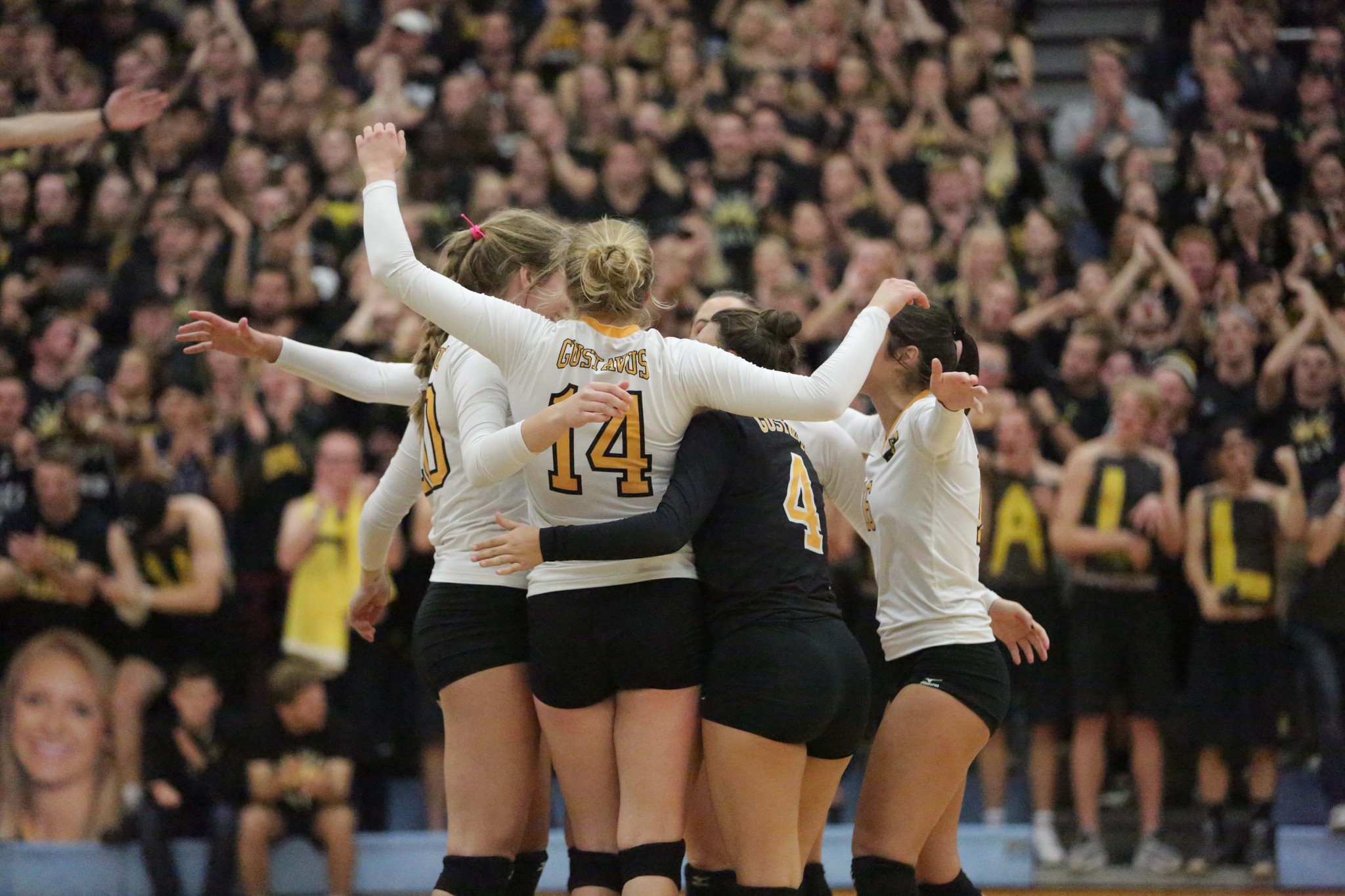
[1134,841,1182,875]
[1070,839,1110,870]
[1250,850,1274,877]
[1187,848,1217,872]
[1031,824,1066,865]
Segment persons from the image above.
[1176,423,1307,878]
[0,628,130,842]
[1047,378,1185,875]
[0,0,1345,896]
[469,306,872,896]
[827,297,1012,896]
[353,120,932,896]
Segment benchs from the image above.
[0,750,1345,896]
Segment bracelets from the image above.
[98,108,113,132]
